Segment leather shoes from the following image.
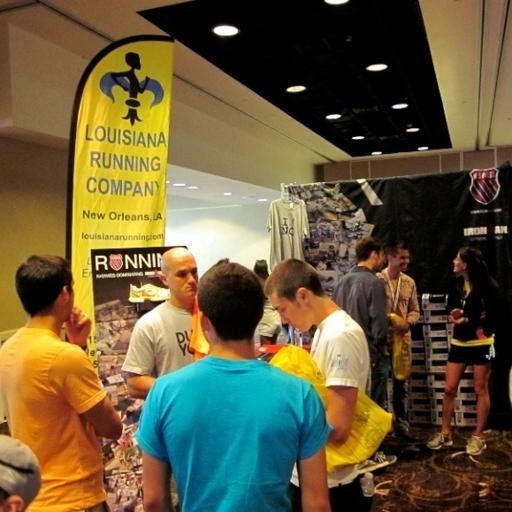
[382,426,427,448]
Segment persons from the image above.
[122,245,202,399]
[0,253,124,512]
[369,240,423,443]
[134,257,340,512]
[249,259,285,347]
[332,241,389,410]
[426,244,498,457]
[264,259,374,511]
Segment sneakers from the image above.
[425,431,454,451]
[465,435,487,456]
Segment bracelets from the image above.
[78,346,88,351]
